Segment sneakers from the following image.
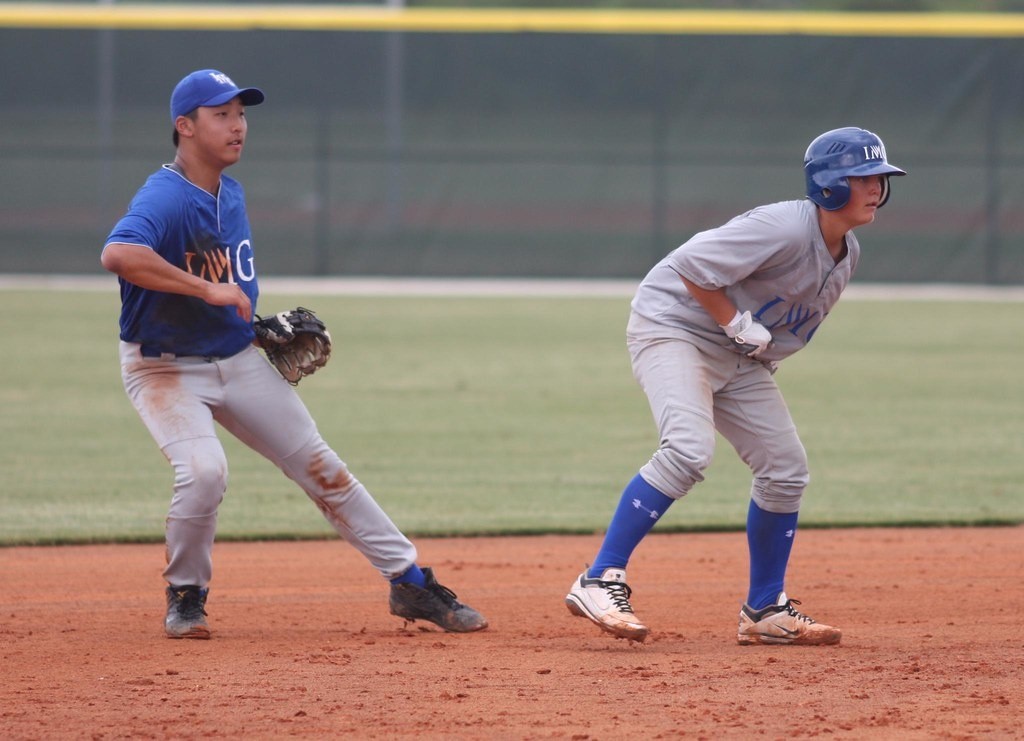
[164,585,211,639]
[389,567,488,633]
[735,591,842,645]
[566,569,647,639]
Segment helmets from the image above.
[804,127,906,210]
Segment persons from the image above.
[564,127,905,642]
[99,69,489,639]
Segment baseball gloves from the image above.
[251,306,332,383]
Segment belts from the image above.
[140,345,198,358]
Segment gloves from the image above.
[717,311,772,356]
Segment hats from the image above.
[170,69,265,125]
